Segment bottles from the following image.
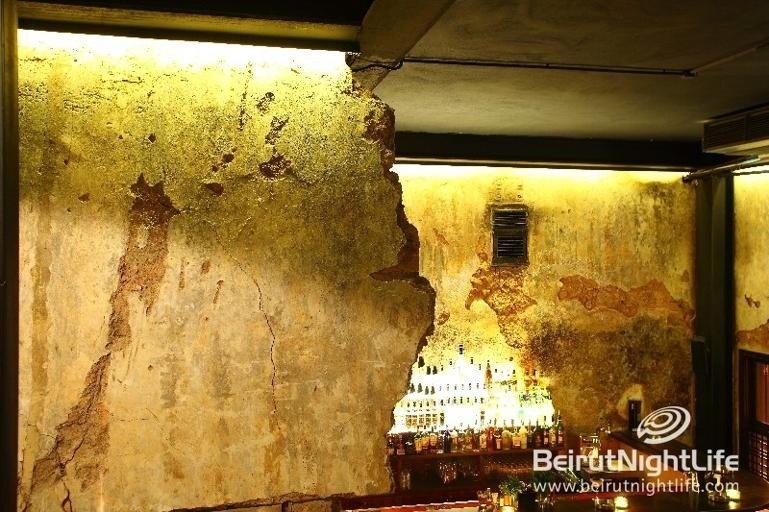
[629,399,642,433]
[476,486,518,512]
[385,339,565,456]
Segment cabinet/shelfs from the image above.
[390,429,580,491]
